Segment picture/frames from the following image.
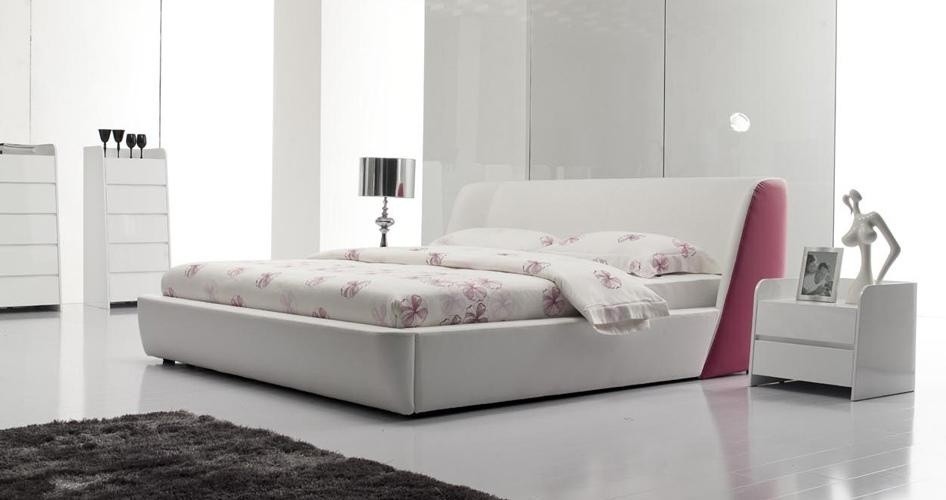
[797,247,846,303]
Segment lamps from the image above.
[359,156,416,247]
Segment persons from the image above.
[803,262,832,295]
[806,255,818,274]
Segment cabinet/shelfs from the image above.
[83,146,171,309]
[0,143,61,313]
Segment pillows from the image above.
[544,230,721,277]
[428,229,555,255]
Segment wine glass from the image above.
[112,129,125,157]
[137,134,147,158]
[97,129,112,157]
[125,133,136,157]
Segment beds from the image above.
[137,178,787,420]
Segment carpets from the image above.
[0,411,505,499]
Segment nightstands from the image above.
[746,269,916,402]
